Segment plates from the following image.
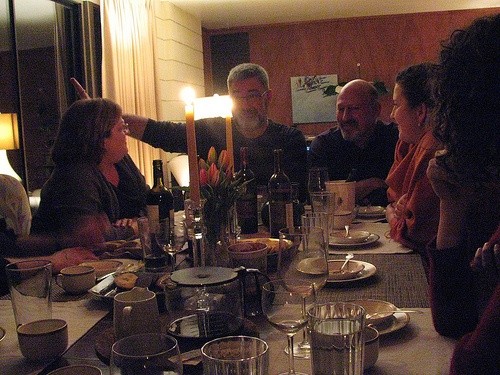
[88,271,142,302]
[356,204,388,219]
[78,261,123,279]
[326,229,379,247]
[234,234,289,259]
[298,256,377,285]
[344,299,410,339]
[0,326,7,341]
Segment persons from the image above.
[427,13,500,375]
[0,98,159,298]
[70,63,308,206]
[307,80,399,209]
[384,62,441,253]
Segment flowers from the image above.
[195,145,229,186]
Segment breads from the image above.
[114,273,139,289]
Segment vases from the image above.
[204,207,236,267]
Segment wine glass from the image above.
[259,166,341,375]
[234,147,259,234]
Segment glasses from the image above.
[113,123,128,135]
[229,90,268,102]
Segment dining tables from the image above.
[0,223,456,375]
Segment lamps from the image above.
[0,114,19,150]
[225,117,233,173]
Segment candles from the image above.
[185,105,202,207]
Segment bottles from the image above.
[146,158,175,265]
[266,150,295,242]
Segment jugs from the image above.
[162,266,274,343]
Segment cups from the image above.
[113,288,159,342]
[46,365,105,375]
[307,301,380,375]
[323,179,356,212]
[56,266,95,293]
[200,336,271,375]
[331,208,353,230]
[228,243,267,278]
[16,318,68,362]
[109,333,184,375]
[136,217,171,273]
[5,259,53,331]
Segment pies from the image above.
[237,238,289,253]
